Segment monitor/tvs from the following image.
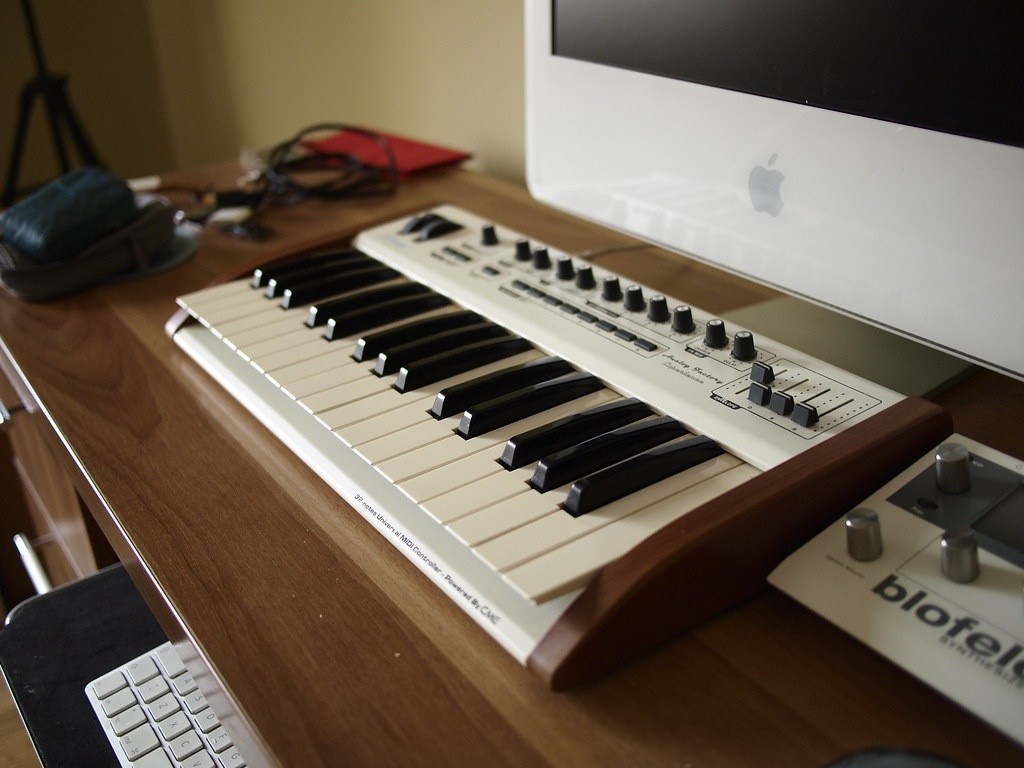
[519,0,1024,403]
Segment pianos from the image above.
[163,199,954,697]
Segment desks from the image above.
[0,157,1024,768]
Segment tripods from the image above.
[0,0,104,210]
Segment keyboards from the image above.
[85,639,248,768]
